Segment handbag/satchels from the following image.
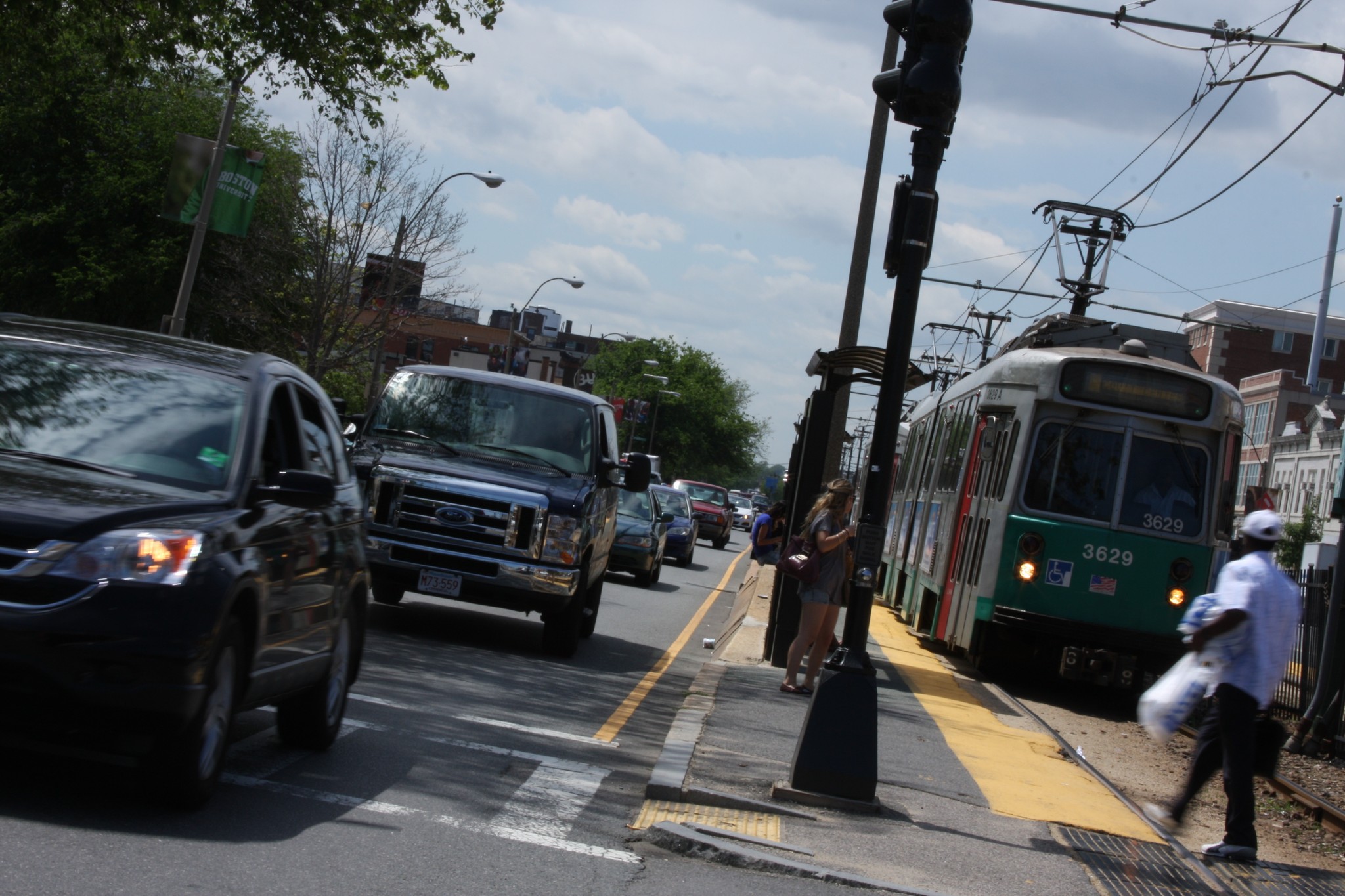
[1255,711,1286,779]
[1137,649,1214,742]
[777,535,822,584]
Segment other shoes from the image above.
[1144,802,1180,835]
[801,685,813,697]
[780,682,801,693]
[1202,840,1258,857]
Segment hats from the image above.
[1240,509,1282,540]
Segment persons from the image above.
[749,501,784,566]
[772,476,857,695]
[1137,510,1304,861]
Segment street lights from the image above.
[628,373,670,451]
[363,171,507,423]
[648,389,683,452]
[505,276,586,372]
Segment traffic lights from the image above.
[871,0,974,126]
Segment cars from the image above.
[608,473,675,585]
[674,478,737,549]
[0,315,381,801]
[726,491,769,534]
[643,480,704,568]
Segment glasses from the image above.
[850,497,856,503]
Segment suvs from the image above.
[341,363,652,664]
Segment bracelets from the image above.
[842,530,846,541]
[836,534,841,544]
[844,528,849,537]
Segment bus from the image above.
[876,199,1248,696]
